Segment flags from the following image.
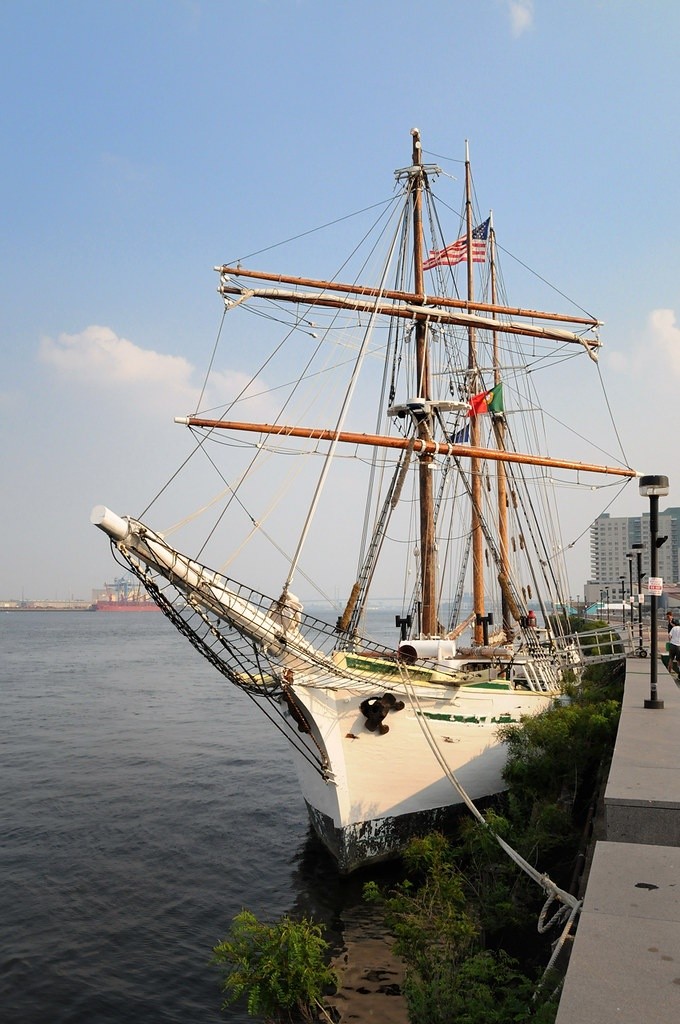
[422,217,491,271]
[450,424,469,442]
[469,383,504,416]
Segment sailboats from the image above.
[89,124,640,881]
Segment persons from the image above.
[666,612,680,672]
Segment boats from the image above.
[90,572,165,613]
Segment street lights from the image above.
[600,589,604,622]
[605,586,610,623]
[569,596,574,616]
[619,575,628,630]
[625,553,635,639]
[631,542,647,657]
[577,594,581,620]
[638,474,671,709]
